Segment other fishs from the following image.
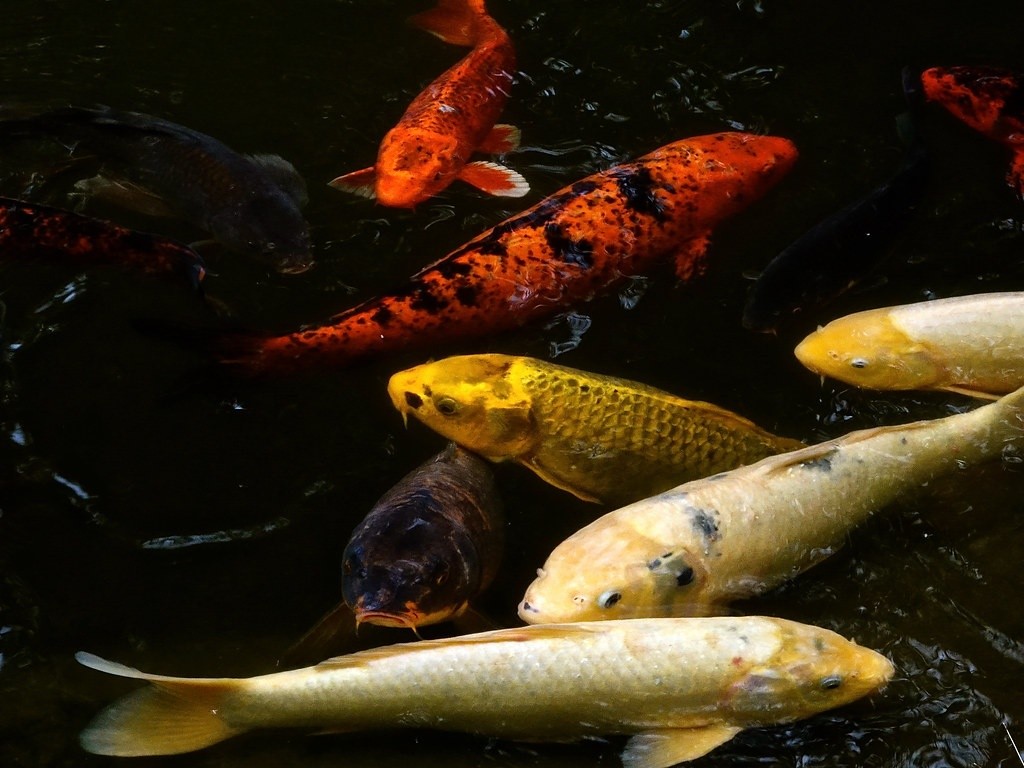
[0,0,1024,767]
